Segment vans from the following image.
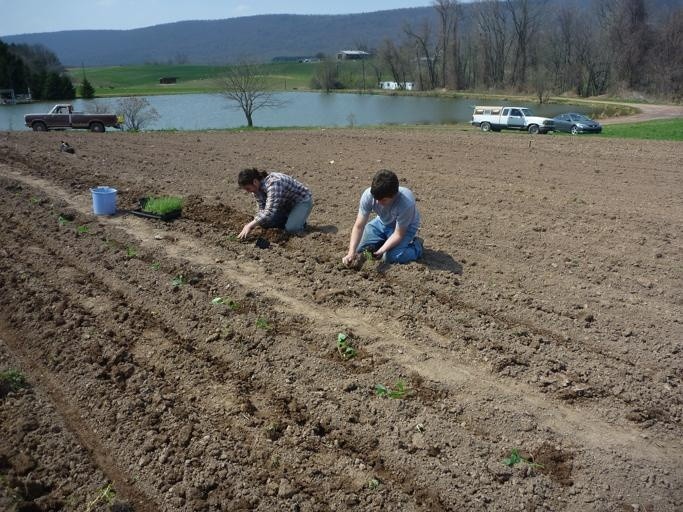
[472,106,555,134]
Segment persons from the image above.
[235,166,314,242]
[340,167,425,270]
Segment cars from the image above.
[554,113,602,134]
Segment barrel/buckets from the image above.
[90,186,118,216]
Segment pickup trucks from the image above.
[25,104,117,132]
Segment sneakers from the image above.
[416,236,424,248]
